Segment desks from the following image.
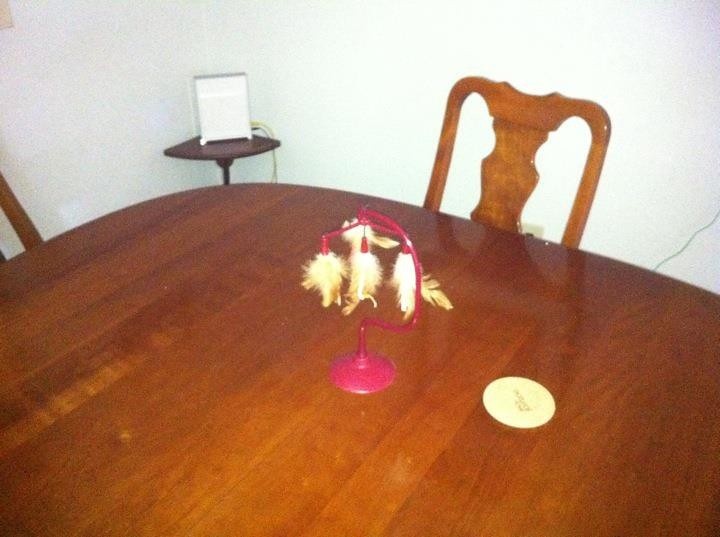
[0,183,719,537]
[164,134,281,184]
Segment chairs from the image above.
[424,77,610,250]
[1,173,44,266]
